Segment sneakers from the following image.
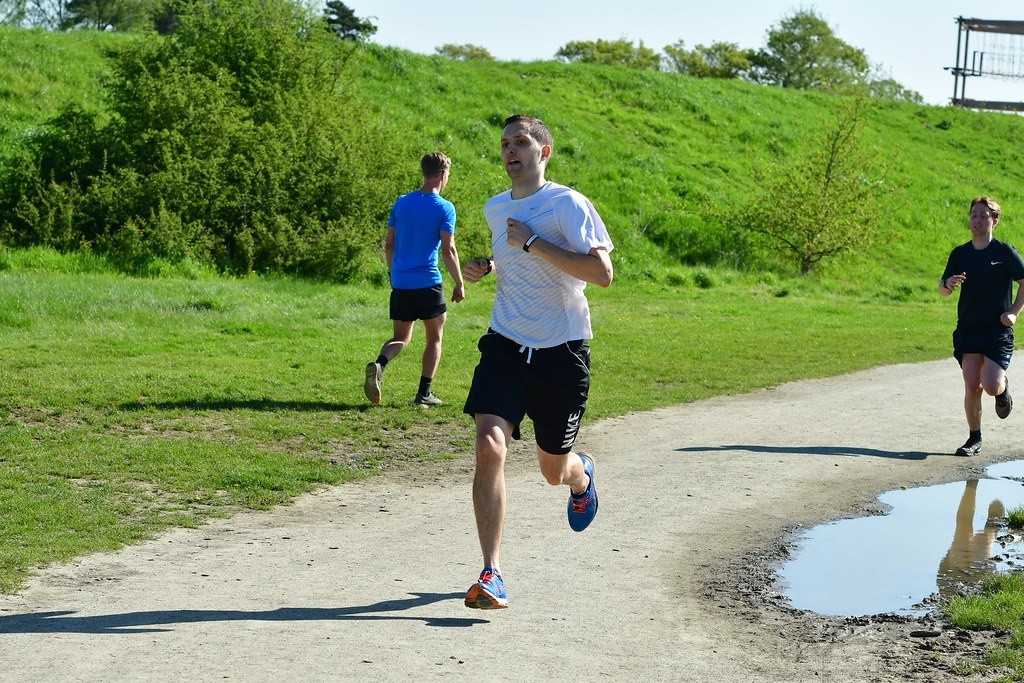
[414,391,443,405]
[956,438,983,456]
[567,453,599,532]
[996,377,1013,419]
[465,566,508,610]
[364,362,383,404]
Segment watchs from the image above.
[942,280,949,289]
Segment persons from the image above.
[364,152,465,407]
[939,198,1024,456]
[464,114,615,609]
[936,480,1004,591]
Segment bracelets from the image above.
[479,258,492,275]
[523,234,539,252]
